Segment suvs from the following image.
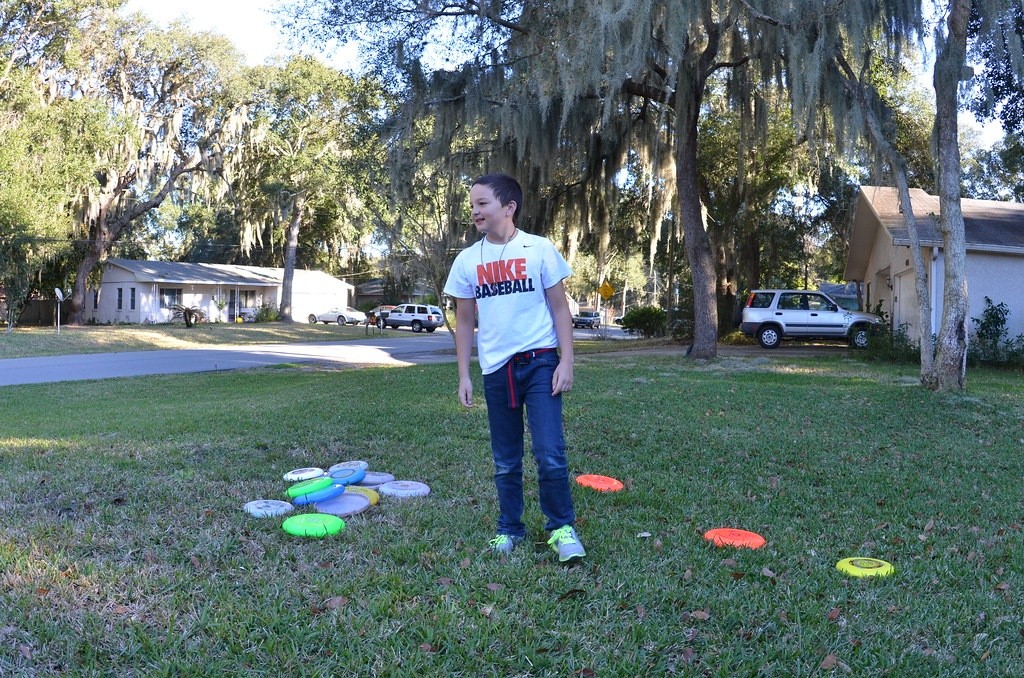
[376,303,444,333]
[573,312,601,329]
[739,288,884,350]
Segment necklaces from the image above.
[481,228,516,291]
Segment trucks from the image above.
[820,293,864,311]
[229,301,261,318]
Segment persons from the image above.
[444,174,586,563]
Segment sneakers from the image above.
[547,525,587,563]
[488,527,527,556]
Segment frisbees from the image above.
[282,460,395,518]
[379,480,431,498]
[836,557,894,578]
[282,513,345,537]
[576,474,624,493]
[243,499,295,518]
[705,528,765,550]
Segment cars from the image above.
[614,317,625,326]
[369,305,398,325]
[308,306,367,326]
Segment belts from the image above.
[504,348,556,408]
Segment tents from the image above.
[818,283,863,311]
